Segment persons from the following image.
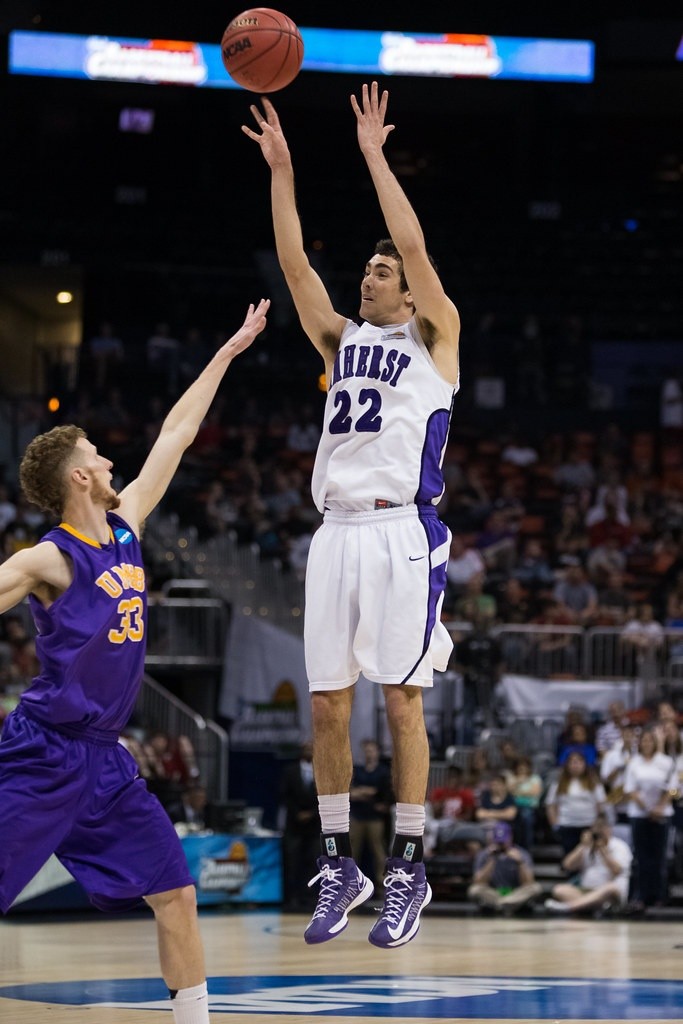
[241,79,463,950]
[1,295,273,1024]
[117,699,683,918]
[543,811,634,918]
[1,387,683,695]
[466,822,543,919]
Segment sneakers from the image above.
[368,857,432,949]
[304,855,374,945]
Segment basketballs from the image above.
[219,6,307,95]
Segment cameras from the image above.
[494,846,506,855]
[592,831,601,840]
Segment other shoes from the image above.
[592,902,612,920]
[622,898,645,915]
[477,905,492,915]
[545,899,567,914]
[500,904,514,918]
